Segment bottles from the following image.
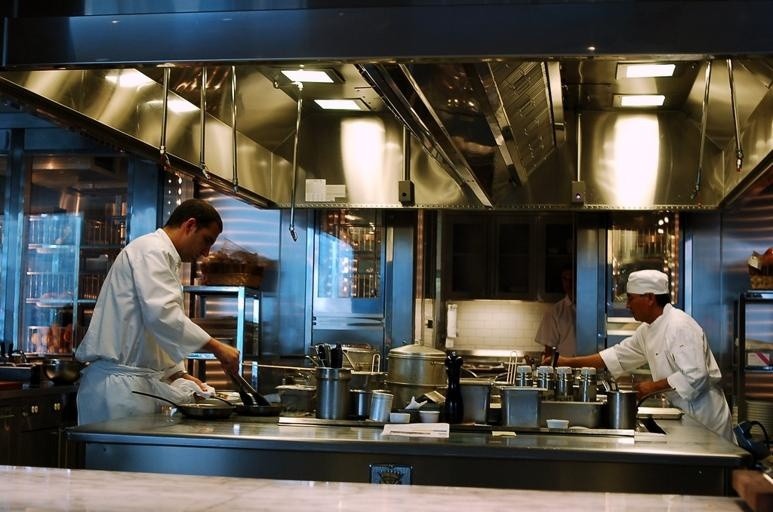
[443,350,463,424]
[538,365,555,401]
[576,366,597,403]
[555,366,575,401]
[516,366,534,387]
[368,392,395,422]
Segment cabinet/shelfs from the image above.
[0,151,15,344]
[731,289,773,445]
[181,285,262,395]
[12,148,135,364]
[331,209,379,298]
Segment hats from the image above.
[626,268,671,295]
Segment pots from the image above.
[310,342,377,371]
[384,339,449,422]
[131,391,234,420]
[350,370,386,391]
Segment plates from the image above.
[744,401,773,437]
[232,402,282,415]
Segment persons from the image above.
[539,270,739,447]
[535,263,577,357]
[75,200,240,427]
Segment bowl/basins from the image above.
[44,360,82,385]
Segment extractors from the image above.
[354,58,567,208]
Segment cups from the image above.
[419,410,441,423]
[607,390,636,429]
[349,389,366,418]
[315,366,352,420]
[389,412,412,424]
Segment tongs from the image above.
[226,368,270,405]
